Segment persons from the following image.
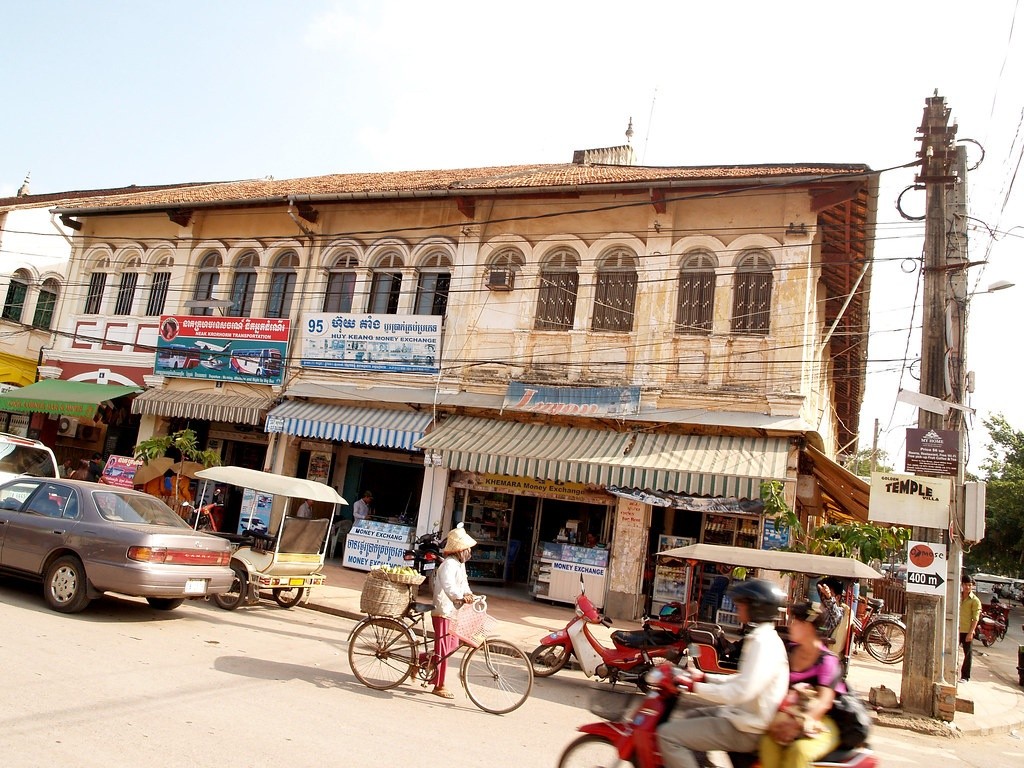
[58,460,72,478]
[85,452,110,485]
[142,475,166,499]
[410,527,478,699]
[297,499,314,519]
[169,474,192,520]
[353,491,373,521]
[957,575,982,683]
[811,578,844,639]
[758,603,847,768]
[658,578,790,768]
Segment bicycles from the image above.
[346,595,533,714]
[841,590,907,664]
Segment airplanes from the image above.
[194,341,232,371]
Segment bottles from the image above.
[764,528,781,543]
[660,544,678,552]
[656,567,686,600]
[465,566,481,578]
[720,595,737,612]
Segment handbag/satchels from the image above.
[447,597,496,648]
[772,683,818,747]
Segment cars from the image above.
[0,477,235,613]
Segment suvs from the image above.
[0,432,59,503]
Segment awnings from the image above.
[264,400,433,451]
[413,414,790,501]
[807,443,896,529]
[131,388,275,425]
[0,378,144,420]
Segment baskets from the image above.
[586,683,638,722]
[360,574,414,618]
[372,567,425,586]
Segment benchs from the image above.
[686,599,723,644]
[201,530,250,546]
[819,603,855,661]
[253,516,329,555]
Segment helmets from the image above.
[659,600,685,622]
[727,578,788,621]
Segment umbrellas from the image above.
[133,457,175,485]
[169,461,206,479]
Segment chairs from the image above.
[322,520,352,559]
[34,499,60,517]
[509,539,520,583]
[700,575,743,630]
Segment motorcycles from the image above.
[529,543,884,695]
[972,573,1024,647]
[183,466,348,610]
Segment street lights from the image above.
[898,281,1015,723]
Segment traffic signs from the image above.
[906,540,946,596]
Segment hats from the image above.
[443,528,477,553]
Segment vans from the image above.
[240,517,267,533]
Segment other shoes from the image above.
[431,688,453,699]
[410,660,419,679]
[958,678,967,683]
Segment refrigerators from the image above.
[650,534,696,621]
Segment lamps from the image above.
[784,223,807,236]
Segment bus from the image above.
[229,348,281,377]
[158,343,200,370]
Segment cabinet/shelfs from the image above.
[529,541,609,612]
[700,513,762,580]
[456,489,516,586]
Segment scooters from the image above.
[403,521,464,598]
[561,662,878,768]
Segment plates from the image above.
[388,521,403,524]
